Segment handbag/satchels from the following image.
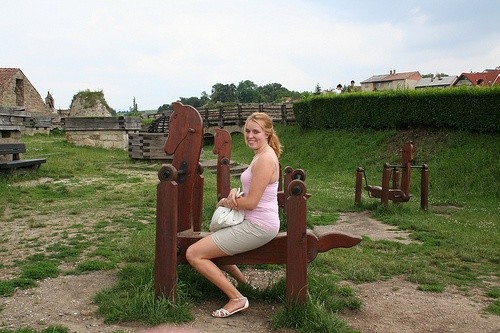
[208,187,245,232]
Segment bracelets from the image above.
[221,198,225,207]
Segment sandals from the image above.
[211,297,249,317]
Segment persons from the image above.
[348,81,357,93]
[186,113,285,318]
[333,84,342,94]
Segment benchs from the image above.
[0,143,47,176]
